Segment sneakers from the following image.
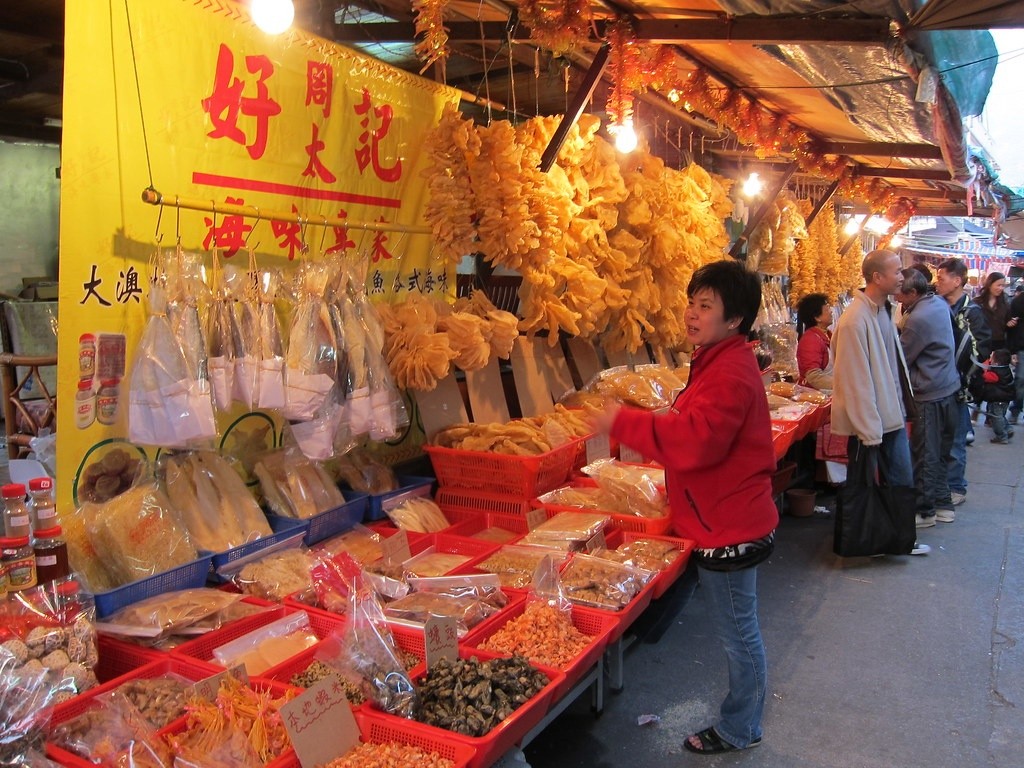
[909,542,930,554]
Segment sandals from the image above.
[684,725,761,754]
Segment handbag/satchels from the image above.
[832,448,917,557]
[950,304,989,371]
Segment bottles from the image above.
[1,484,32,538]
[0,525,71,600]
[29,478,60,531]
[75,333,125,431]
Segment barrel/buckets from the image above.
[787,488,817,517]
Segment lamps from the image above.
[915,66,937,104]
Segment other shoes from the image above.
[916,510,955,527]
[951,491,966,505]
[971,420,977,427]
[990,430,1014,444]
[984,421,992,427]
[966,431,975,445]
[1008,419,1017,425]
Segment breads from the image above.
[77,449,140,503]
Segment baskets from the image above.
[35,399,832,768]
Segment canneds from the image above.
[0,478,70,602]
[75,333,126,429]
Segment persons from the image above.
[977,348,1015,445]
[893,267,954,527]
[908,263,968,507]
[1014,285,1024,298]
[583,261,779,754]
[796,293,835,391]
[967,272,1018,429]
[1004,291,1024,425]
[1009,353,1018,378]
[828,248,931,556]
[935,258,976,446]
